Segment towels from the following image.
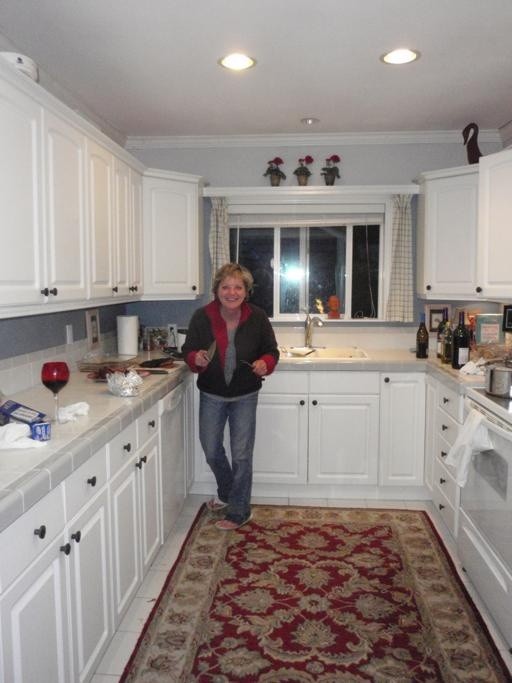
[443,410,495,488]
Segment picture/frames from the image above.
[424,304,452,340]
[84,309,102,351]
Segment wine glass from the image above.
[41,360,70,425]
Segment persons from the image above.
[181,263,280,529]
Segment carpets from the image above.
[117,499,512,683]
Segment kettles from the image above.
[176,328,187,354]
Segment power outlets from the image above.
[168,322,179,346]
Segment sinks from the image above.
[277,345,370,361]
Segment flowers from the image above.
[261,151,342,186]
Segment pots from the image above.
[484,358,512,399]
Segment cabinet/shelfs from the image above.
[1,437,111,681]
[0,55,87,320]
[454,509,512,679]
[409,163,476,301]
[143,166,205,302]
[192,366,381,497]
[427,374,464,568]
[380,365,428,498]
[87,123,143,309]
[476,147,511,306]
[108,389,160,631]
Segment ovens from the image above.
[458,398,512,571]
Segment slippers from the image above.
[206,498,229,512]
[215,512,254,530]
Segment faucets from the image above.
[299,307,325,348]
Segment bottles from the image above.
[416,313,430,359]
[437,306,471,368]
